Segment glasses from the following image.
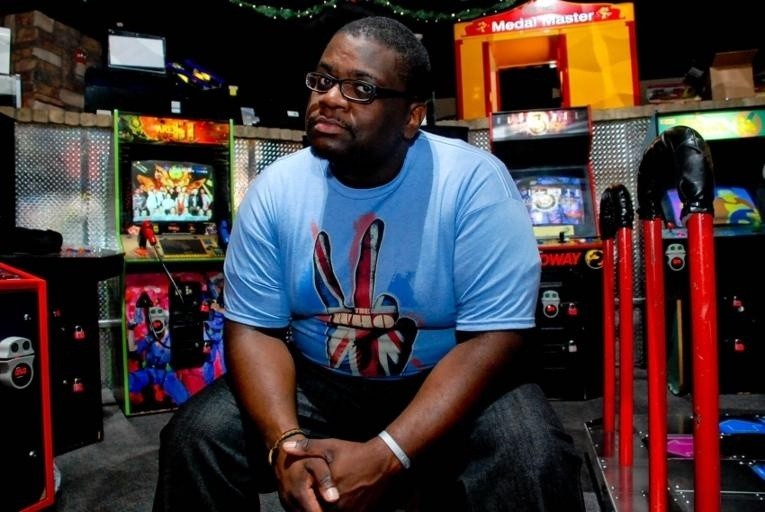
[305,69,419,108]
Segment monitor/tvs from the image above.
[661,179,765,229]
[507,163,597,239]
[120,148,226,233]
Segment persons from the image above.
[125,311,191,407]
[195,272,229,386]
[146,16,589,508]
[133,183,209,216]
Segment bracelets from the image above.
[266,426,314,468]
[379,429,415,471]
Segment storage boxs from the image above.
[706,47,761,99]
[2,11,101,111]
